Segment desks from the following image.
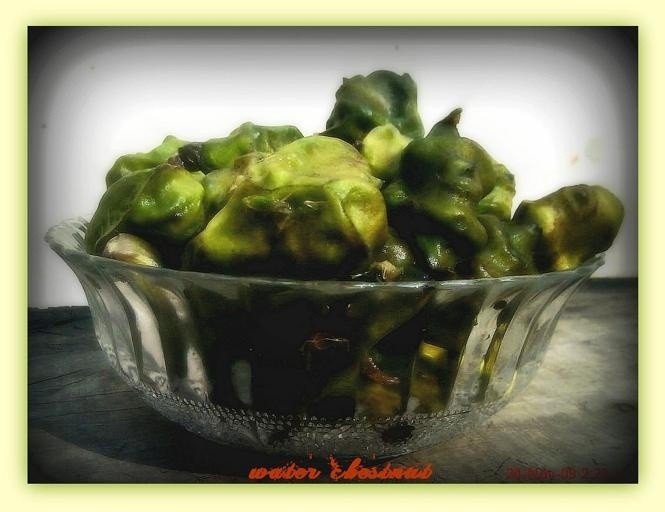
[29,273,640,486]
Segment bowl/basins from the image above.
[42,211,608,464]
